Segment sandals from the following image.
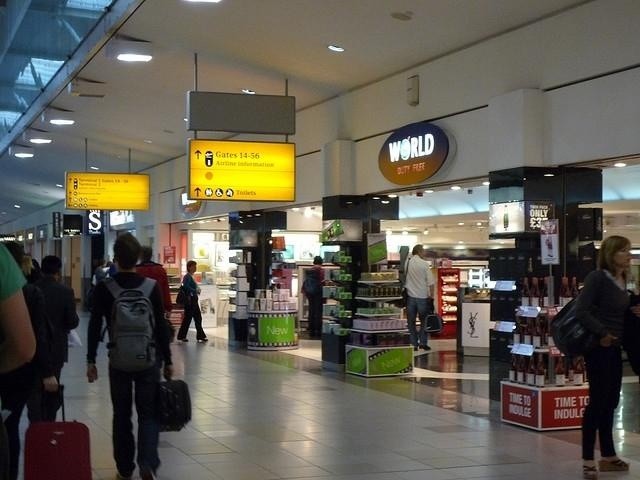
[598,457,630,473]
[582,462,599,479]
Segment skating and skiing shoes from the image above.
[414,344,431,351]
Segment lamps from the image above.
[40,108,76,125]
[104,40,152,62]
[66,78,106,98]
[9,144,35,159]
[22,129,56,145]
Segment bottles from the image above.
[559,276,578,308]
[547,232,553,259]
[569,358,588,386]
[508,274,550,388]
[504,205,508,228]
[555,357,566,387]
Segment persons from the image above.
[135,245,171,317]
[177,260,209,343]
[34,255,79,382]
[1,242,56,479]
[91,259,116,292]
[0,244,36,372]
[403,244,436,351]
[83,234,177,480]
[573,234,640,480]
[19,254,40,284]
[300,255,327,340]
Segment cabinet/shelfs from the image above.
[437,268,461,340]
[502,306,591,433]
[344,279,416,376]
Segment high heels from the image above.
[177,335,189,342]
[196,336,209,344]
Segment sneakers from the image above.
[116,465,137,480]
[138,464,157,480]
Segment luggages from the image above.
[24,383,92,480]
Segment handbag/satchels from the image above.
[424,313,444,333]
[177,286,189,303]
[157,379,192,431]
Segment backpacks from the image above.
[549,288,600,358]
[303,271,320,296]
[103,277,158,374]
